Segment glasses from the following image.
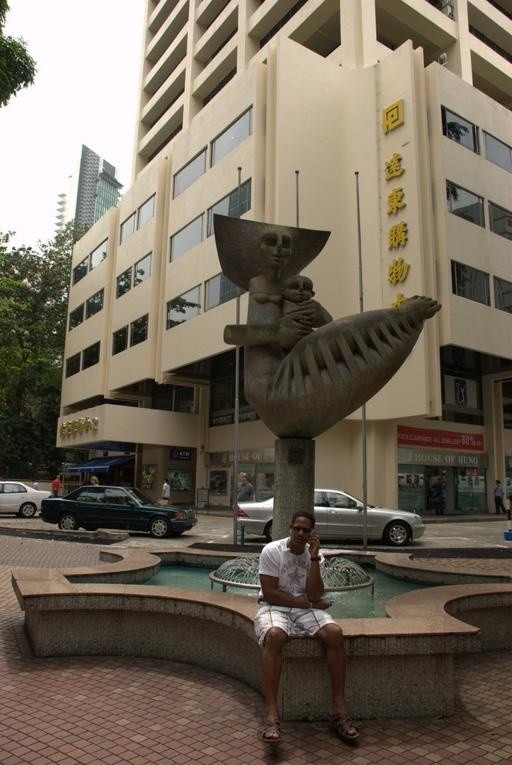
[292,525,313,533]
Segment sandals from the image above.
[327,711,360,740]
[261,719,282,742]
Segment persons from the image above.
[276,274,318,345]
[251,510,361,744]
[232,471,257,520]
[493,480,507,515]
[50,474,61,497]
[90,475,101,486]
[245,223,297,307]
[505,494,512,521]
[222,311,313,353]
[31,478,39,491]
[425,477,445,514]
[161,477,171,502]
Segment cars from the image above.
[37,482,200,540]
[235,486,444,550]
[0,480,54,519]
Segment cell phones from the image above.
[311,527,320,535]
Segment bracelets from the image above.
[308,601,314,608]
[309,555,322,561]
